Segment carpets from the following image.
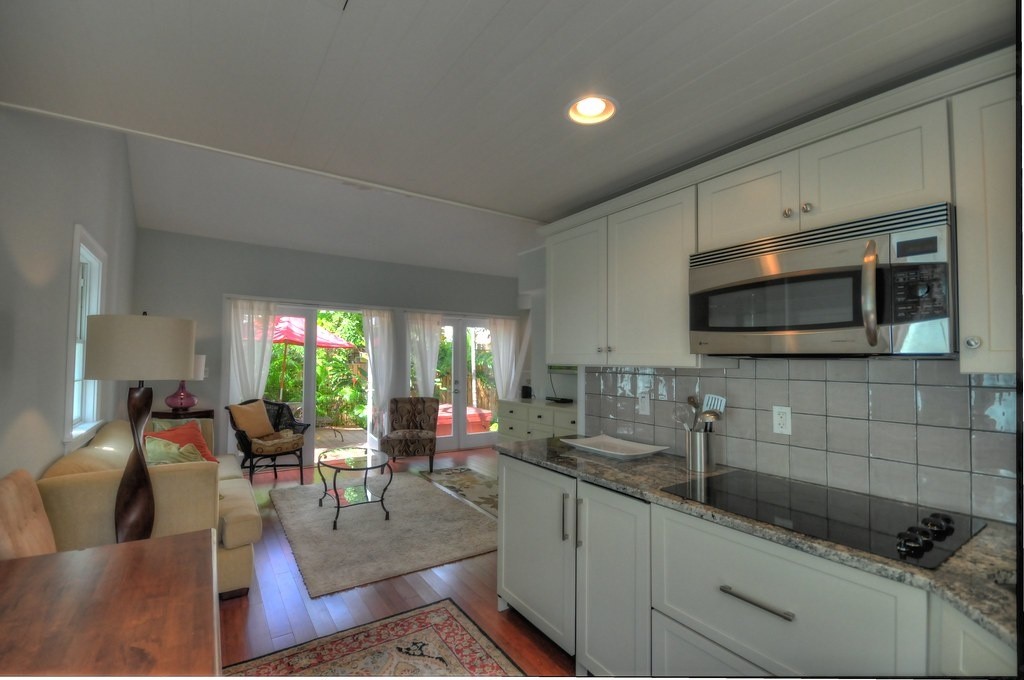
[221,597,530,677]
[420,467,498,517]
[268,471,499,598]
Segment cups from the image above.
[686,431,716,471]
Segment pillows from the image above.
[144,419,220,467]
[228,398,275,439]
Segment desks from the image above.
[1,525,222,677]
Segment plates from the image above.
[560,434,670,461]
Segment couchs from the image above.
[39,418,263,604]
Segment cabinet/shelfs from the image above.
[543,184,738,372]
[495,399,576,443]
[695,96,949,253]
[494,454,652,677]
[960,72,1019,374]
[651,502,1018,677]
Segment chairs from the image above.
[316,397,346,442]
[229,399,312,485]
[379,396,440,473]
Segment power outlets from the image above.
[773,406,792,435]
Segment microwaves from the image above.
[688,203,959,356]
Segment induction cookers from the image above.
[659,468,987,573]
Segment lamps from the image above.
[83,309,194,544]
[165,353,204,411]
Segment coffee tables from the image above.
[317,446,393,531]
